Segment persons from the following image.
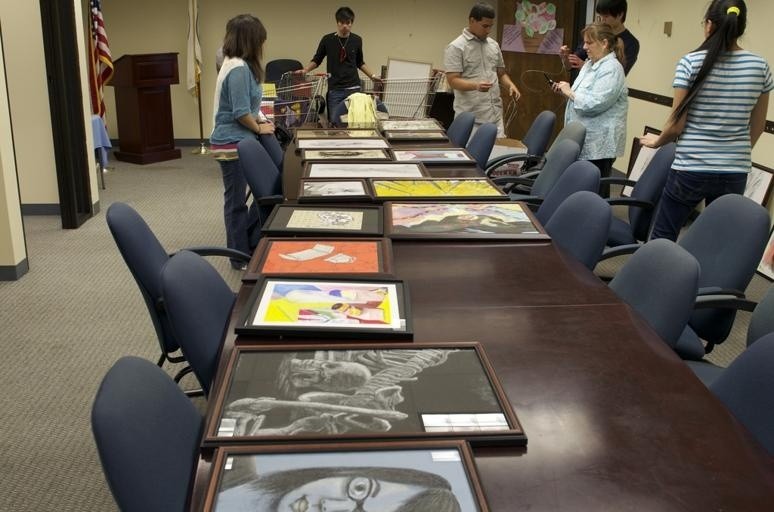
[560,0,639,76]
[546,21,628,196]
[209,13,276,271]
[443,3,521,140]
[214,457,461,511]
[297,7,383,123]
[638,1,773,240]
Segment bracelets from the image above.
[371,74,376,80]
[257,126,261,135]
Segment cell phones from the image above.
[544,73,561,92]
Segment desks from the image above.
[189,245,774,512]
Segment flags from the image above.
[186,0,203,105]
[88,0,114,130]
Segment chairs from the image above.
[486,120,678,284]
[91,355,206,512]
[708,332,774,451]
[104,200,252,399]
[160,249,240,392]
[677,194,774,354]
[265,58,325,126]
[606,235,701,348]
[681,286,774,387]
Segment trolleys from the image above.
[258,70,332,136]
[357,68,453,126]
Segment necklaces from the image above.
[336,32,350,63]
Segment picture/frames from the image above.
[200,341,527,450]
[234,272,414,339]
[261,109,555,239]
[201,439,492,512]
[381,57,433,119]
[242,237,394,284]
